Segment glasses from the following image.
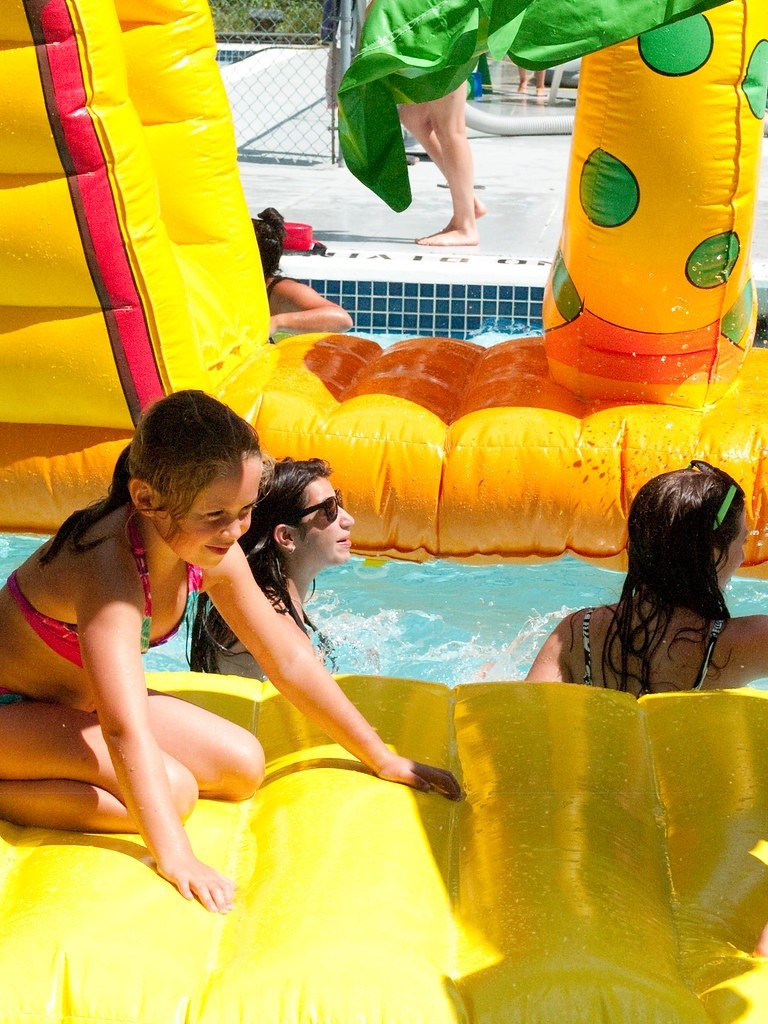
[290,488,344,524]
[686,459,746,531]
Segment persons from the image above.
[0,389,462,917]
[526,460,768,697]
[399,76,486,247]
[518,66,546,96]
[250,208,354,344]
[185,456,355,680]
